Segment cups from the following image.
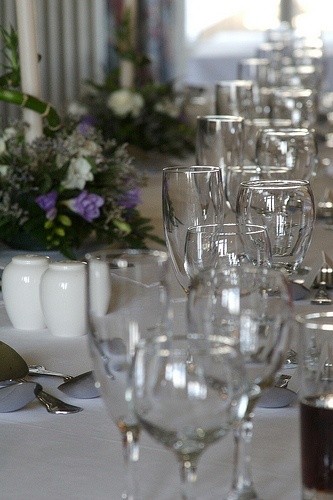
[298,312,333,500]
[1,251,112,339]
[190,37,326,212]
[160,164,224,294]
[237,179,316,280]
[226,165,289,219]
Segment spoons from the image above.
[0,340,107,417]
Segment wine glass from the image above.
[86,224,284,500]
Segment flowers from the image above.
[0,115,167,260]
[81,69,196,159]
